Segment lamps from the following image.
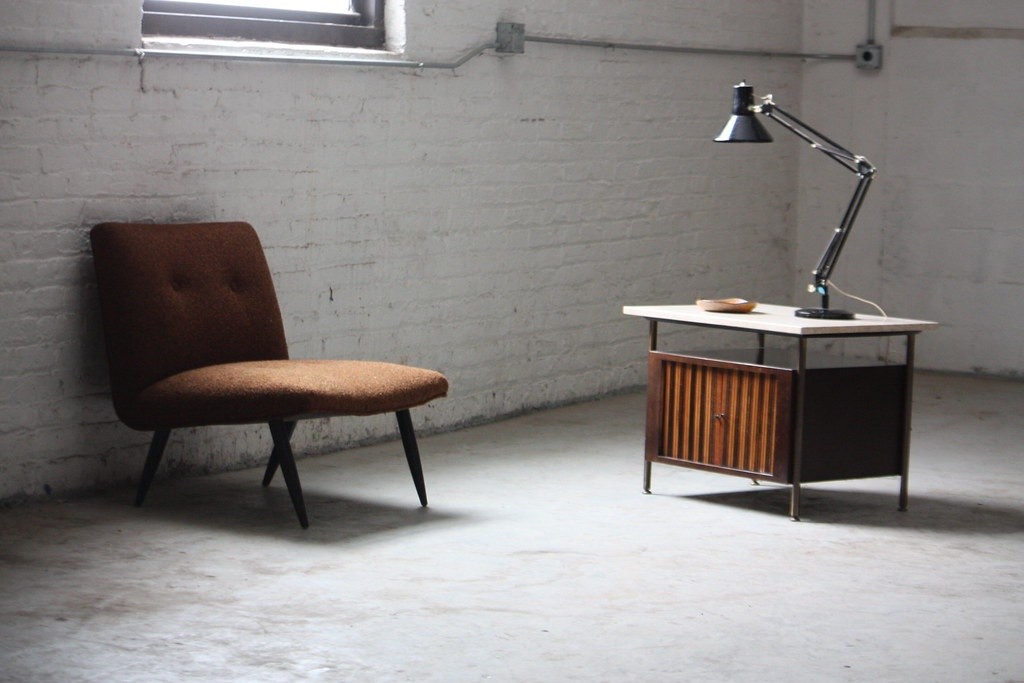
[712,78,882,320]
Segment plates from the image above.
[696,298,756,312]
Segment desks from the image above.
[619,300,942,525]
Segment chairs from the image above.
[89,221,454,529]
